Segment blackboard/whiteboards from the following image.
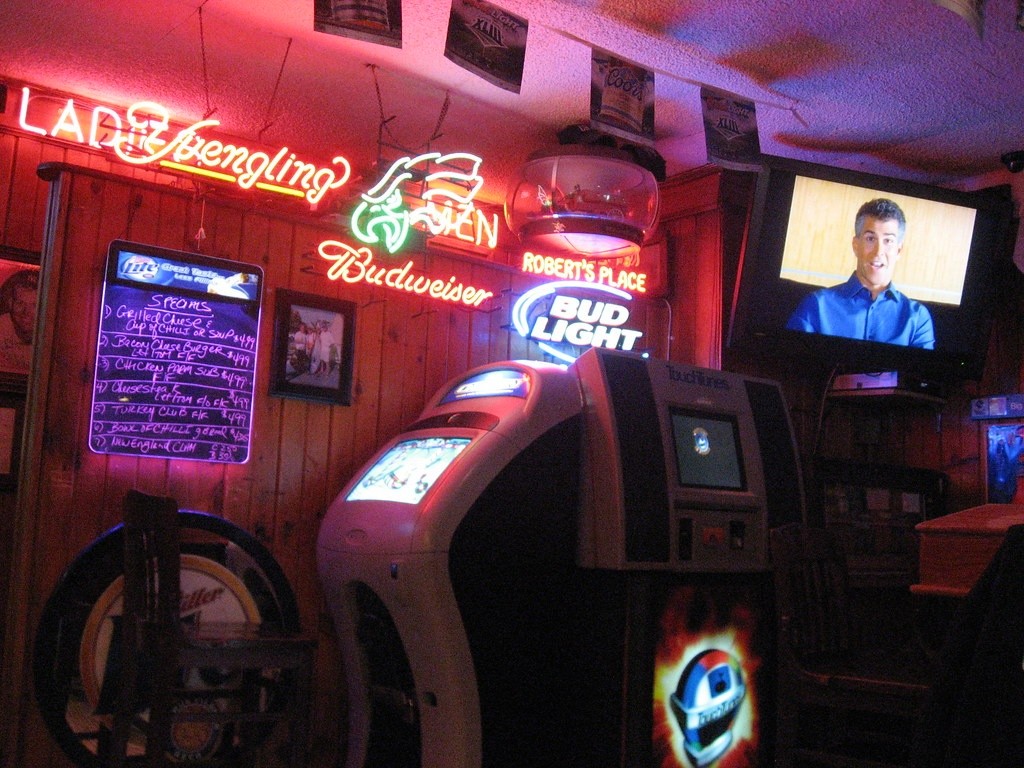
[88,239,265,465]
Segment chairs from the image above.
[769,518,943,768]
[107,488,318,768]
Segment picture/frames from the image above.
[267,286,356,408]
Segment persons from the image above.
[0,270,40,372]
[784,198,936,350]
[294,323,336,376]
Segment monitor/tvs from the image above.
[664,404,748,495]
[344,437,476,505]
[725,153,1016,395]
